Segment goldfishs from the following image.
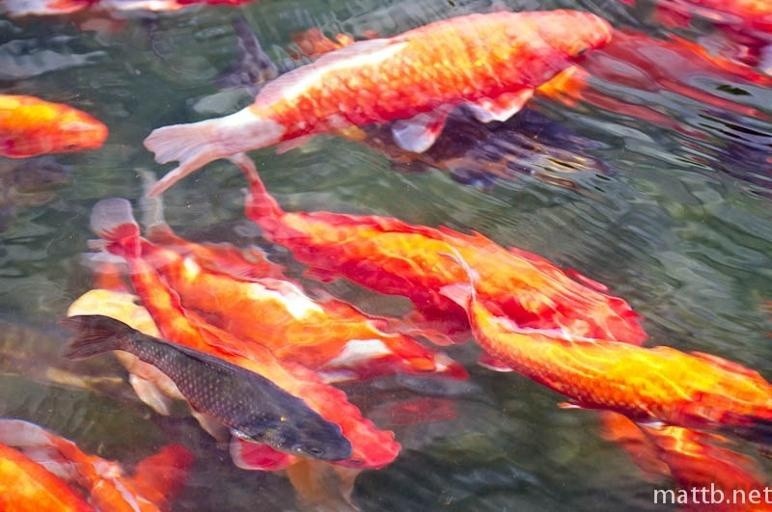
[0,2,771,511]
